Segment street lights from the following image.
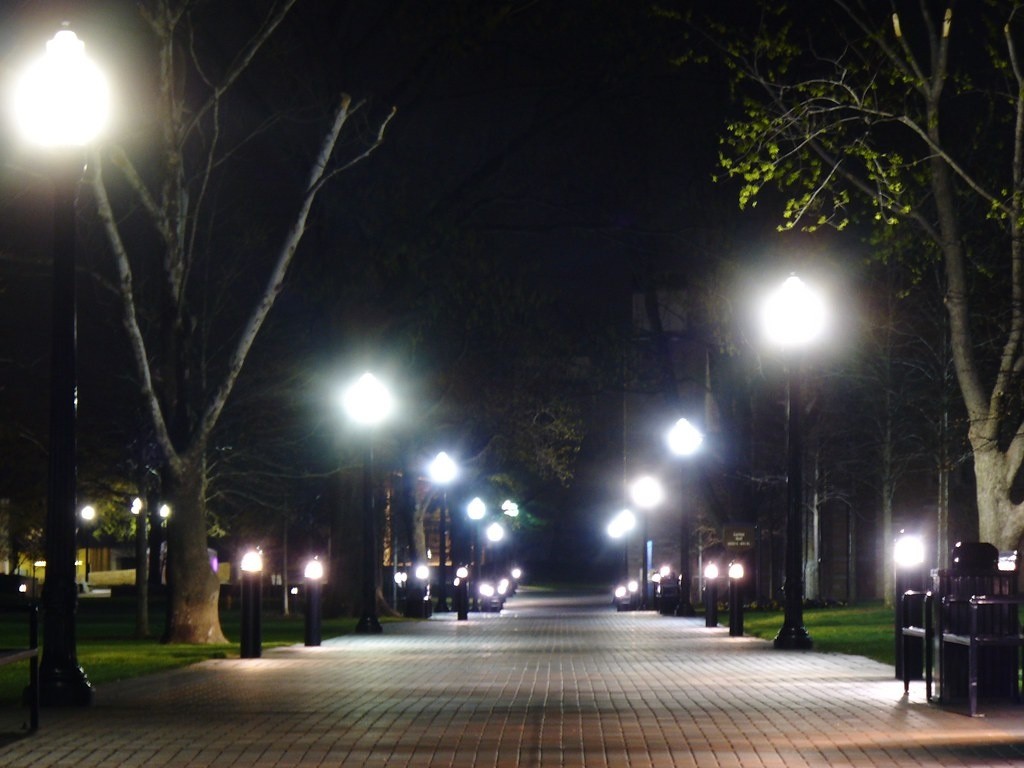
[606,509,634,605]
[487,522,505,595]
[428,452,458,612]
[666,403,704,615]
[763,276,826,651]
[81,506,95,582]
[343,370,391,636]
[467,496,487,612]
[631,478,663,611]
[9,32,113,719]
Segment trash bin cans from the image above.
[938,569,1019,716]
[406,581,432,620]
